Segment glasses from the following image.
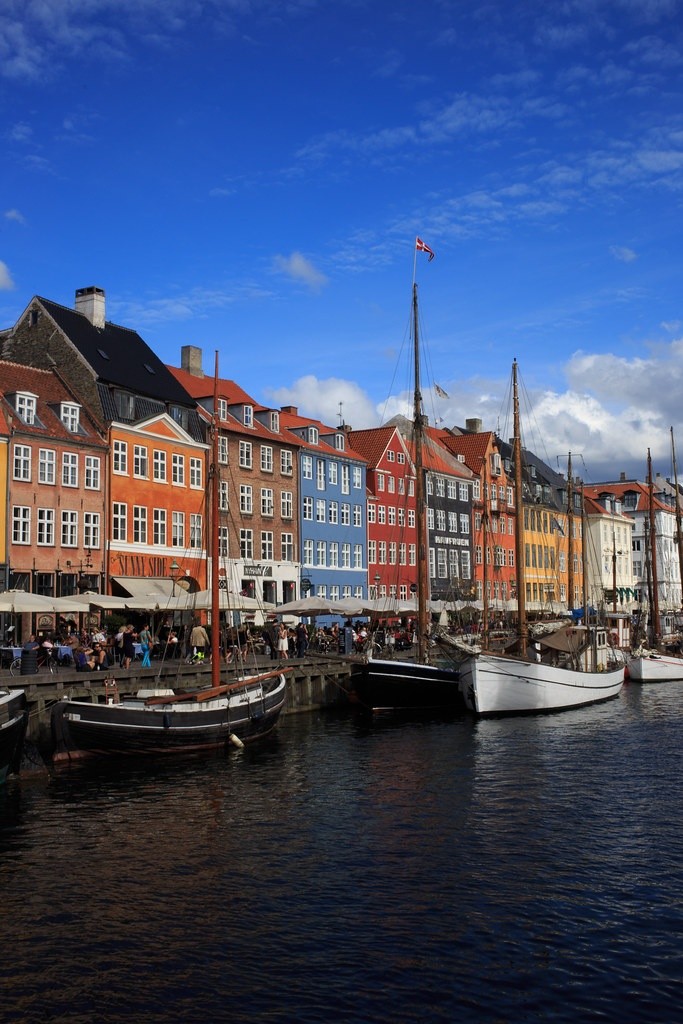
[95,646,100,648]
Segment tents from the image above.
[3,580,571,618]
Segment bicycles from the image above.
[8,648,59,676]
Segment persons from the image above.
[22,613,508,673]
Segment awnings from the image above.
[112,571,189,599]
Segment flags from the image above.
[416,238,434,262]
[434,381,450,399]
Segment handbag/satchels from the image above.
[148,641,153,650]
[118,635,123,648]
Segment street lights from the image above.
[169,561,178,626]
[373,571,380,599]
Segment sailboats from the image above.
[52,350,292,755]
[458,357,625,716]
[348,280,464,715]
[627,448,683,682]
[0,689,28,749]
